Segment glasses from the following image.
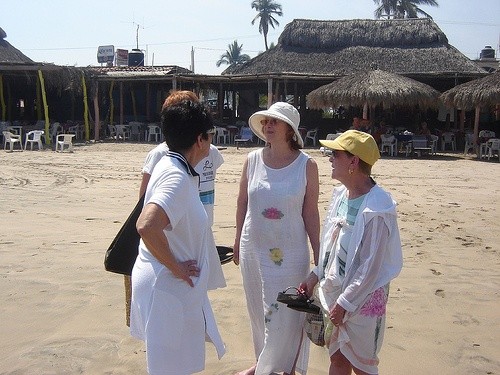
[259,119,279,126]
[331,150,337,158]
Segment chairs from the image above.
[0,118,500,163]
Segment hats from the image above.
[248,102,304,149]
[319,130,380,166]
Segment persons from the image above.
[139,89,225,228]
[296,130,403,375]
[129,99,227,375]
[416,121,430,135]
[233,101,320,375]
[352,117,387,145]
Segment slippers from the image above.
[276,286,320,315]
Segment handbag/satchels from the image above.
[305,311,325,348]
[104,192,146,275]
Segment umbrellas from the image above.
[441,70,500,107]
[306,69,440,120]
